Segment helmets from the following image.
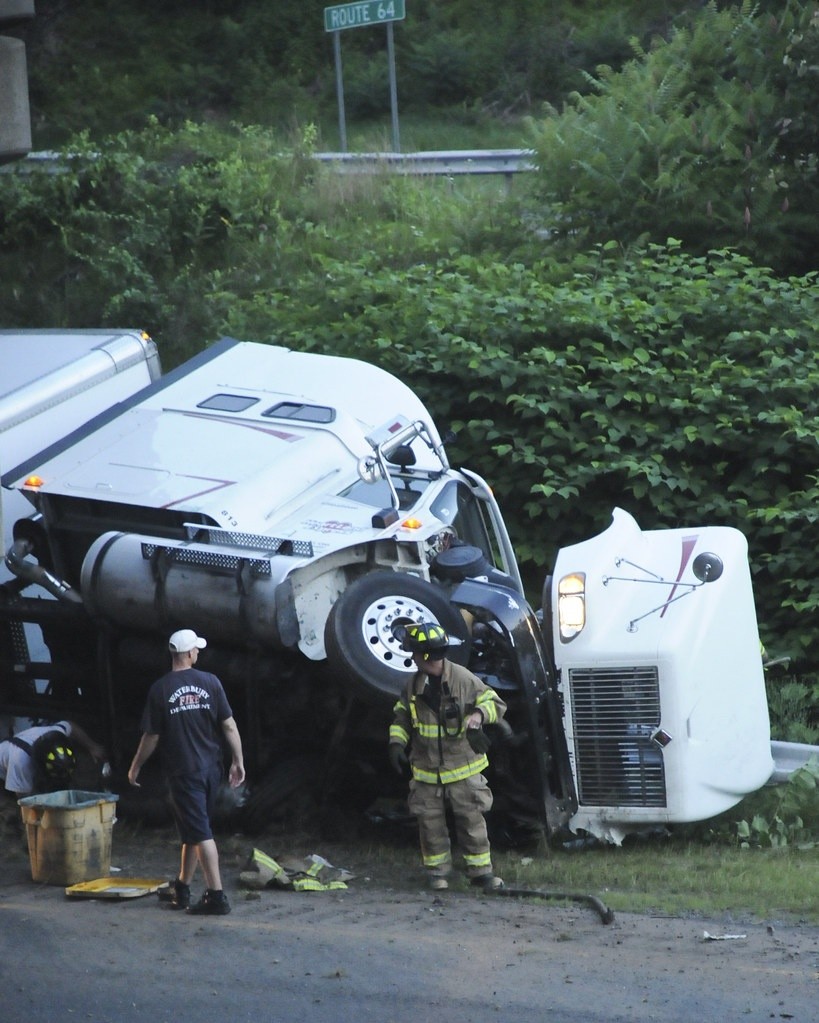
[45,745,77,780]
[399,622,449,661]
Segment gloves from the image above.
[388,743,409,775]
[466,725,491,754]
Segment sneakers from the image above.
[184,888,231,915]
[157,876,190,910]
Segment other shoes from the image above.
[471,874,504,889]
[424,876,448,890]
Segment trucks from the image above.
[0,328,819,851]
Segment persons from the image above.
[129,629,244,914]
[0,720,107,850]
[389,623,508,892]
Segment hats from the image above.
[169,629,207,653]
